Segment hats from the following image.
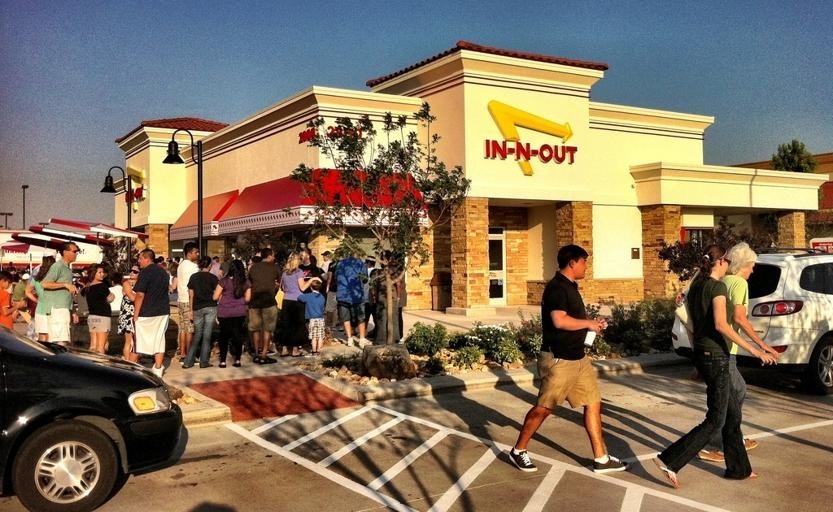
[321,251,333,257]
[22,273,30,282]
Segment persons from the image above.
[117,265,140,361]
[177,241,201,363]
[696,241,781,462]
[27,249,56,340]
[156,256,168,271]
[391,249,407,345]
[182,255,218,369]
[280,250,323,357]
[212,259,251,368]
[653,247,779,489]
[334,248,373,349]
[326,248,346,331]
[81,263,115,354]
[508,244,637,473]
[320,251,333,282]
[1,271,27,332]
[297,280,325,356]
[41,241,80,348]
[364,255,380,338]
[166,262,179,295]
[132,248,171,379]
[210,256,222,278]
[247,248,282,365]
[11,273,35,328]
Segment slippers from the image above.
[280,353,291,358]
[746,470,760,482]
[652,455,680,491]
[291,352,305,357]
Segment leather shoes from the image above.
[743,438,762,453]
[219,362,226,368]
[232,360,241,367]
[260,358,278,365]
[697,450,726,462]
[252,357,259,363]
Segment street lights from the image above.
[102,168,132,270]
[99,166,131,264]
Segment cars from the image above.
[1,323,183,512]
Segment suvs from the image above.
[670,246,833,394]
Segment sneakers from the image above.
[347,336,354,347]
[151,363,165,379]
[193,357,200,365]
[593,455,633,473]
[508,447,537,473]
[182,364,192,368]
[358,338,373,349]
[178,358,185,365]
[200,363,213,368]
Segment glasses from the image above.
[72,249,80,253]
[191,250,201,254]
[128,269,140,275]
